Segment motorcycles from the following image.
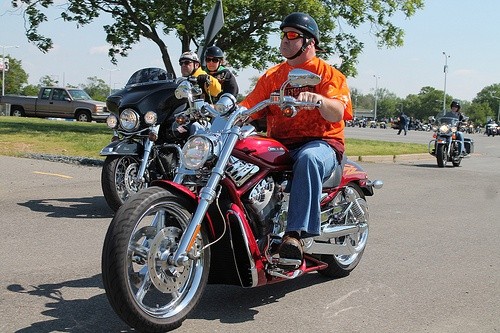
[99,68,267,213]
[101,67,383,333]
[484,120,500,137]
[429,110,475,168]
[343,114,483,135]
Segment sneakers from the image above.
[278,231,303,270]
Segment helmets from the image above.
[280,12,320,47]
[204,46,224,58]
[451,101,460,112]
[179,52,200,63]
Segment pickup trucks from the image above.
[0,85,111,123]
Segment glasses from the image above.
[206,58,223,63]
[180,61,194,66]
[280,31,310,40]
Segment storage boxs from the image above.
[464,137,474,153]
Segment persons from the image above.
[178,52,222,194]
[209,12,353,265]
[202,46,239,108]
[345,112,432,136]
[432,101,468,158]
[466,118,500,137]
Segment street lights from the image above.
[372,74,381,121]
[0,45,19,96]
[442,52,451,115]
